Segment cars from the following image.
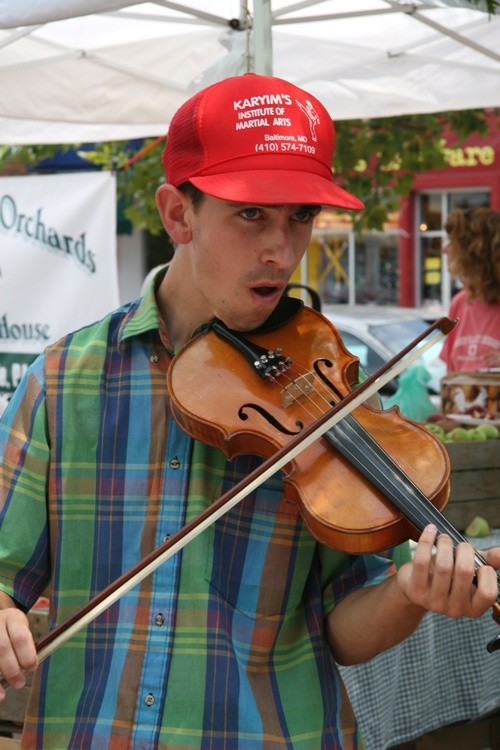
[306,302,448,409]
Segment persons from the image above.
[437,206,500,432]
[0,71,500,750]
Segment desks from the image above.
[335,527,500,750]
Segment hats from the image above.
[162,72,365,211]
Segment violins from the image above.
[167,296,499,654]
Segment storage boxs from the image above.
[389,717,499,750]
